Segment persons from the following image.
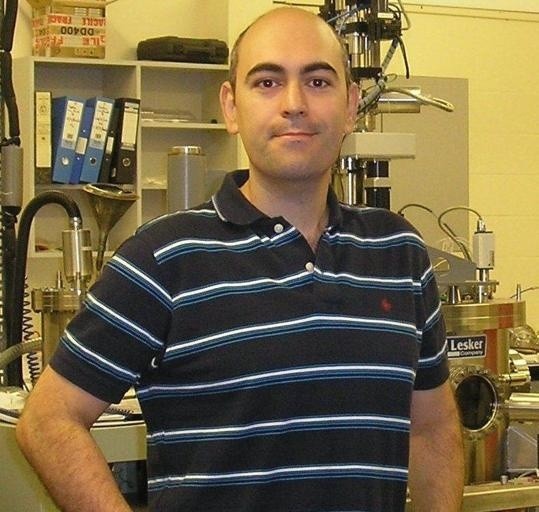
[13,7,467,512]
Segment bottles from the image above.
[166,143,207,210]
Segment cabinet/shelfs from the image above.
[0,52,473,511]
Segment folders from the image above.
[35,91,141,184]
